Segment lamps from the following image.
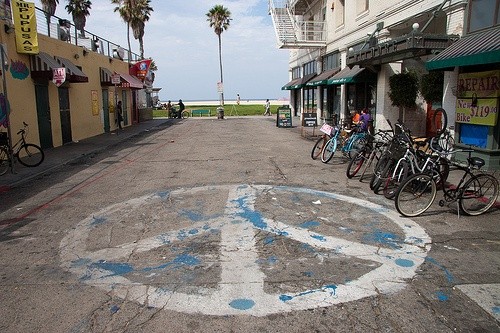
[412,23,420,31]
[348,47,354,53]
[109,59,113,63]
[83,50,89,56]
[4,24,12,34]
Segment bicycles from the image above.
[168,104,189,119]
[310,114,451,199]
[0,121,45,176]
[394,135,500,219]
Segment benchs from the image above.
[191,109,211,117]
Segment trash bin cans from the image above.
[216,107,224,119]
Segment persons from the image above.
[350,110,360,124]
[350,107,370,136]
[263,99,272,116]
[167,101,172,119]
[177,100,185,119]
[115,101,124,129]
[237,94,240,105]
[156,99,167,110]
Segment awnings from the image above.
[282,72,316,90]
[327,65,366,84]
[98,66,120,86]
[426,27,500,72]
[54,56,88,83]
[114,72,143,89]
[306,67,340,86]
[29,52,72,80]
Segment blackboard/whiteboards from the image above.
[302,113,317,127]
